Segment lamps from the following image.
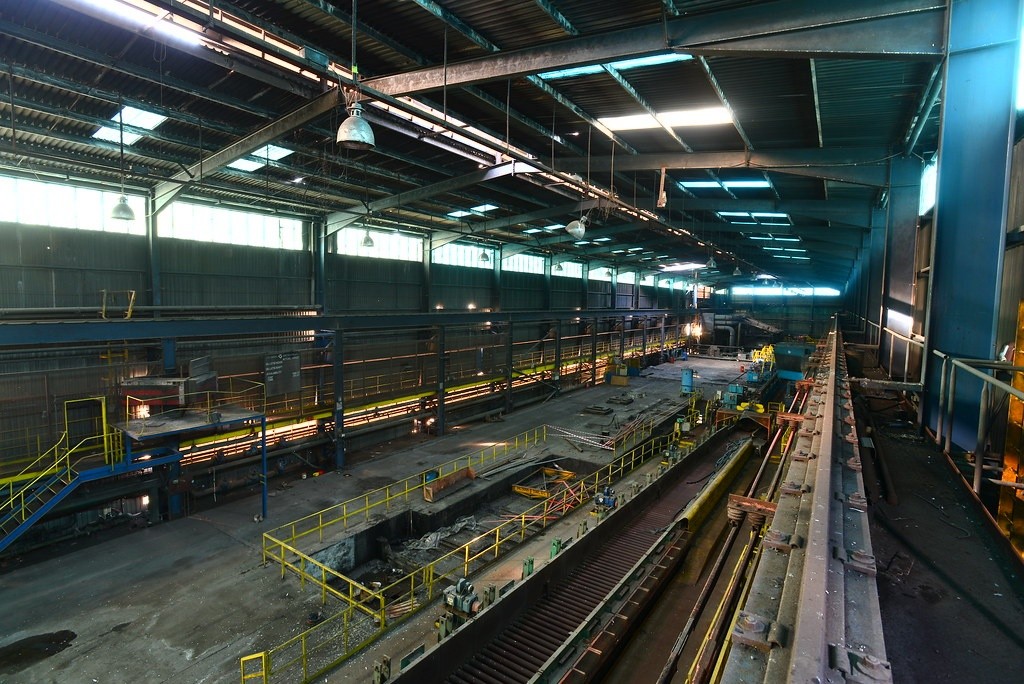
[336,0,374,151]
[478,204,489,261]
[112,95,135,220]
[360,217,374,246]
[565,196,600,240]
